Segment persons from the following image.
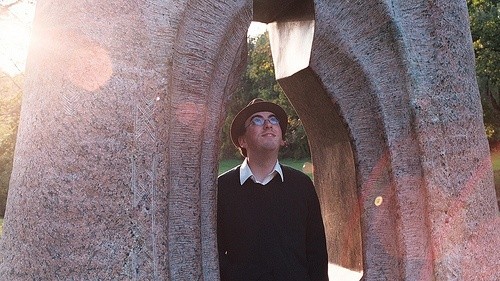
[214,98,330,281]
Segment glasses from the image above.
[245,116,280,129]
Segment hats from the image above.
[230,98,288,148]
